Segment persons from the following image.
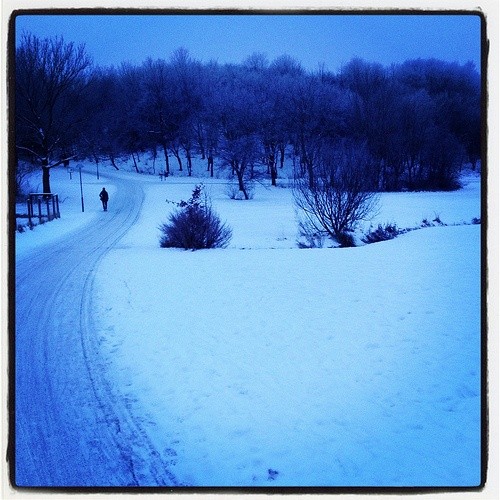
[100,186,109,212]
